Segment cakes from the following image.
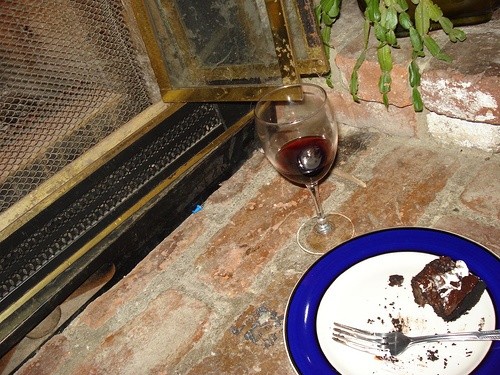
[411,255,487,322]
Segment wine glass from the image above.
[253,83,355,255]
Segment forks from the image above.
[332,322,500,359]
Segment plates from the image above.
[283,226,500,375]
